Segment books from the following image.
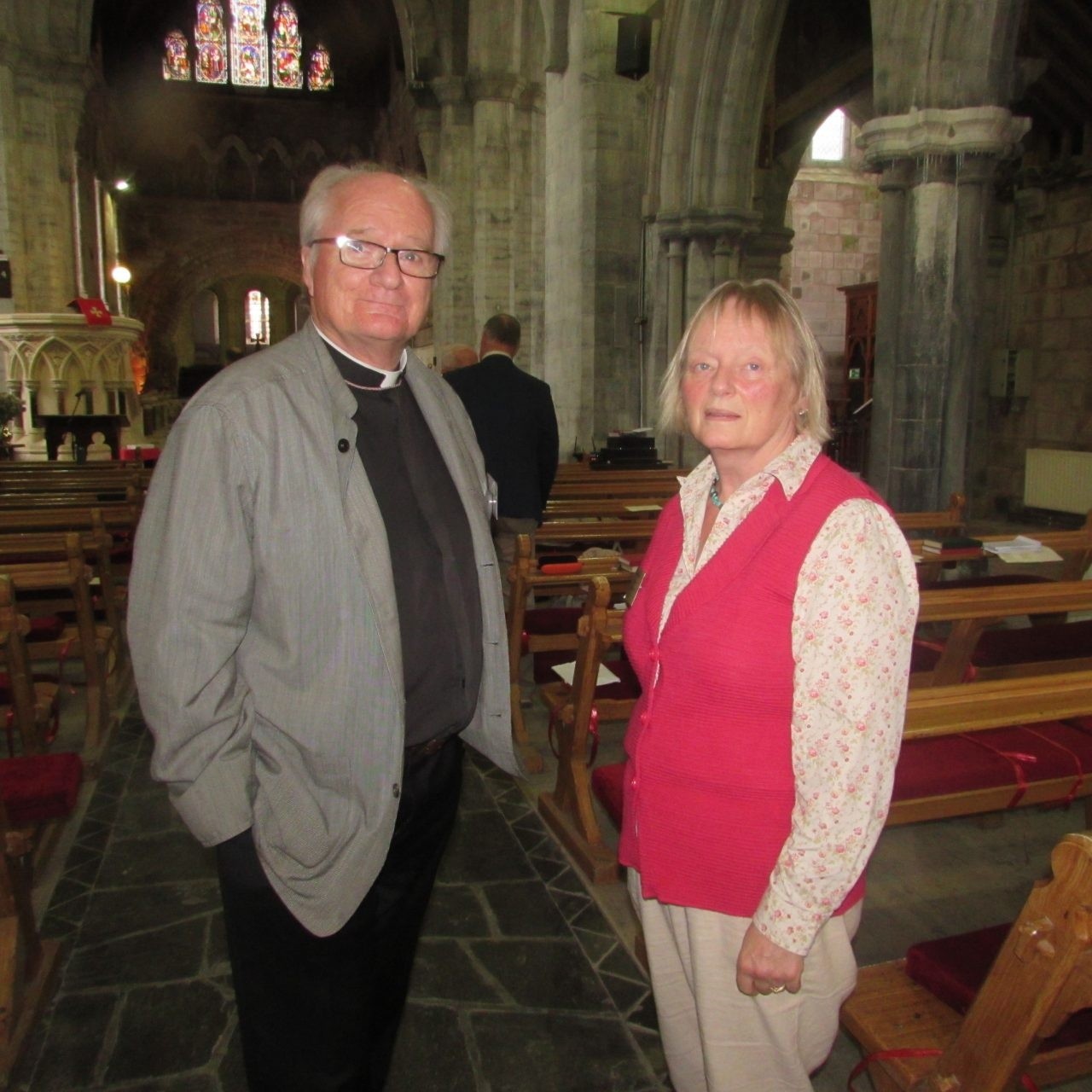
[922,536,1063,564]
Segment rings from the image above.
[770,984,785,994]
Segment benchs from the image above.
[0,459,152,1042]
[481,442,1092,1091]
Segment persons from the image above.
[622,278,918,1092]
[125,165,523,1092]
[445,315,561,610]
[441,343,478,375]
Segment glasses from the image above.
[307,235,445,279]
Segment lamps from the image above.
[112,171,131,193]
[111,254,132,284]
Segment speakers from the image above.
[616,15,652,80]
